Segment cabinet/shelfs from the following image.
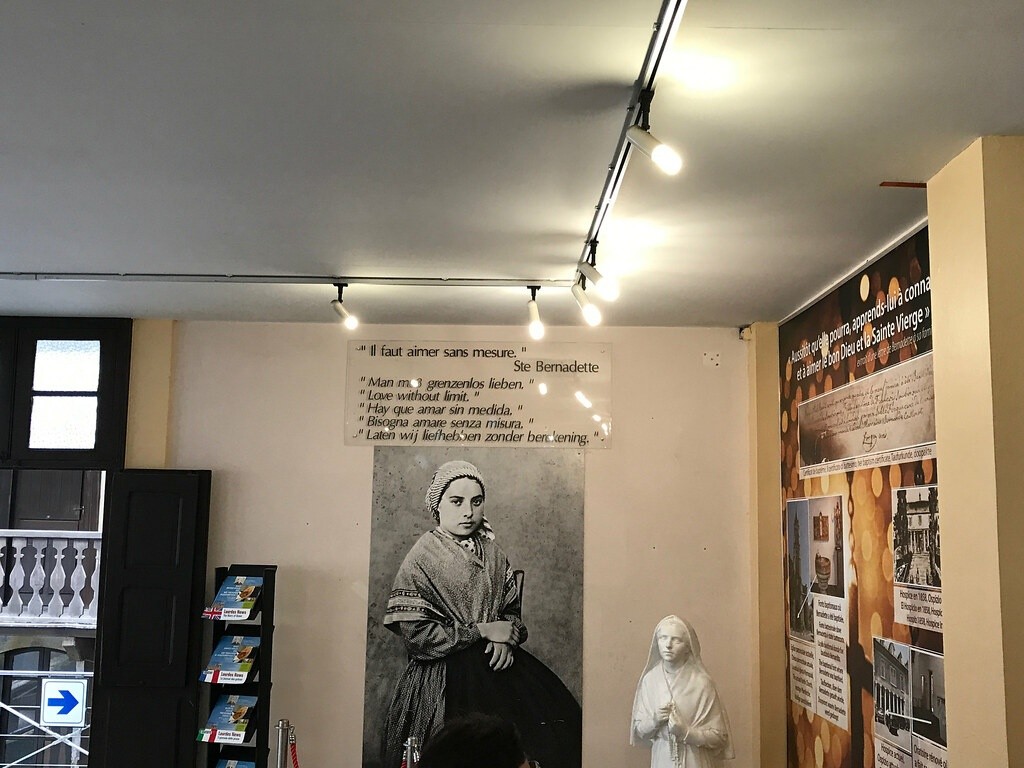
[196,563,279,768]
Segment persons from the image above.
[378,458,582,768]
[629,615,736,768]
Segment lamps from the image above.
[580,240,619,302]
[624,88,683,175]
[526,286,544,340]
[329,282,359,330]
[572,274,602,326]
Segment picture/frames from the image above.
[361,445,586,768]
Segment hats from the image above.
[424,460,486,516]
